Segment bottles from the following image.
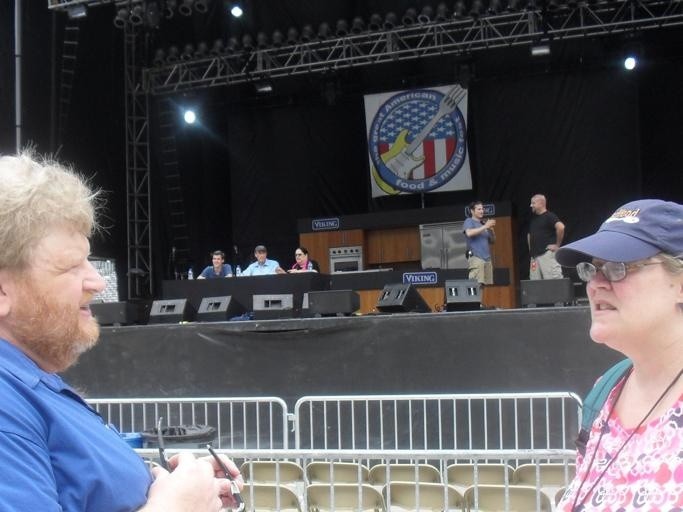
[308,261,312,271]
[235,265,242,276]
[188,269,194,279]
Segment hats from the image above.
[255,245,266,252]
[555,199,682,273]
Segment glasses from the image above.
[575,260,665,283]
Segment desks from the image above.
[161,272,332,322]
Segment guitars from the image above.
[373,86,465,192]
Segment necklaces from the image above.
[569,365,682,512]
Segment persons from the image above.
[274,246,320,274]
[195,250,233,279]
[461,200,497,310]
[0,139,246,511]
[551,198,682,512]
[240,245,280,276]
[525,194,566,308]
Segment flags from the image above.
[362,82,474,200]
